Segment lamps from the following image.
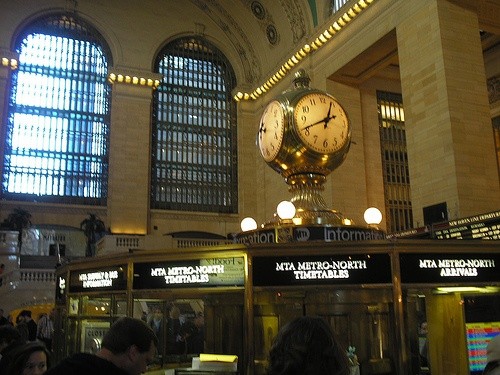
[241,216,258,232]
[364,207,383,231]
[276,200,297,224]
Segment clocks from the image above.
[256,99,285,163]
[294,93,350,154]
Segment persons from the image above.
[42,317,159,375]
[0,305,54,375]
[266,315,350,375]
